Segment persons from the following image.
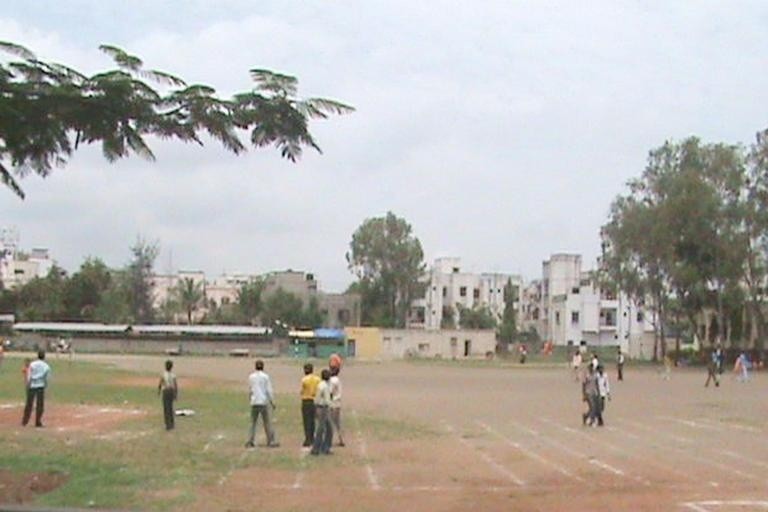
[21,349,51,426]
[565,338,626,426]
[301,354,345,457]
[157,359,178,430]
[704,344,752,388]
[244,359,280,448]
